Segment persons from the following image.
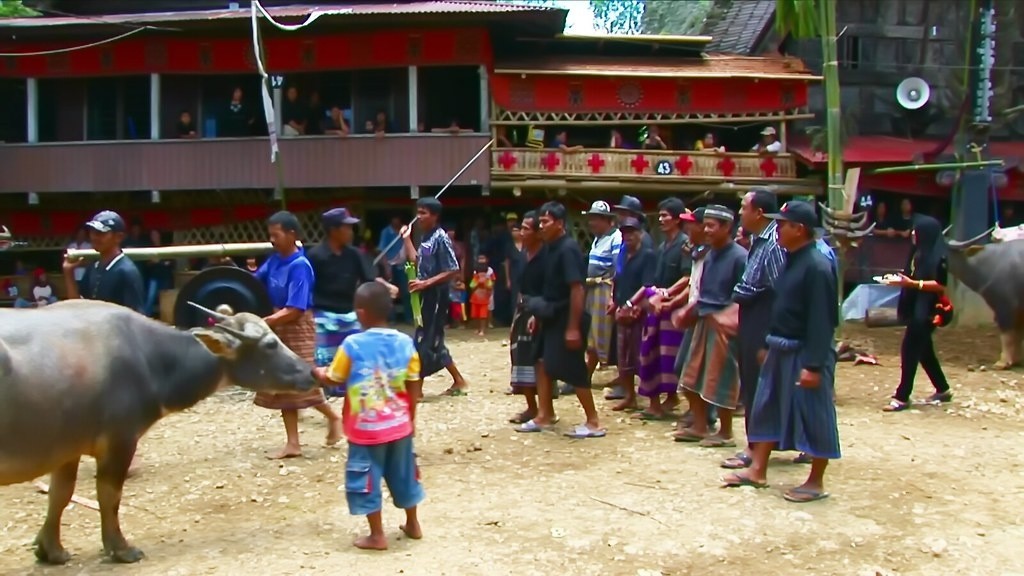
[252,212,345,459]
[792,200,839,465]
[720,189,789,470]
[721,201,843,502]
[515,200,608,438]
[12,83,1024,423]
[311,281,425,550]
[671,204,749,448]
[675,208,718,432]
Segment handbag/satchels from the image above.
[931,294,954,327]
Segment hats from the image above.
[322,208,360,228]
[579,194,818,232]
[86,210,127,232]
[506,212,518,220]
[760,126,776,136]
[33,269,45,277]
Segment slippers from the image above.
[504,374,738,447]
[783,485,830,502]
[882,401,911,411]
[718,471,769,489]
[720,452,752,469]
[923,392,953,402]
[439,387,471,396]
[792,451,813,463]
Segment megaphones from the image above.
[894,76,931,111]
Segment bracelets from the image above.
[626,301,632,308]
[918,280,924,289]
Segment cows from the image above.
[940,223,1024,369]
[0,300,320,563]
